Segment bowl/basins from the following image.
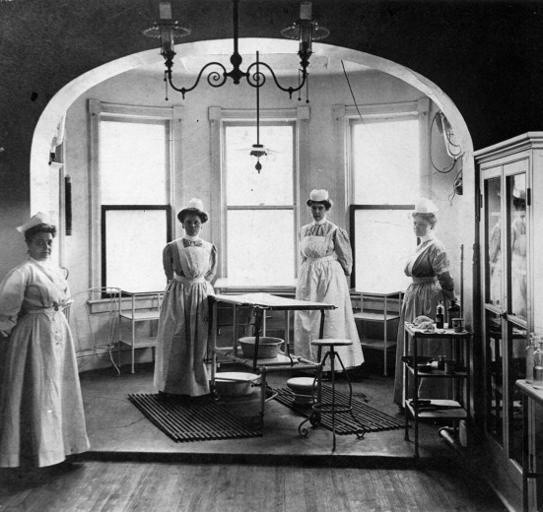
[213,369,262,396]
[234,333,286,359]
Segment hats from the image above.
[512,173,525,199]
[306,189,331,210]
[16,210,56,235]
[177,198,208,224]
[411,198,437,224]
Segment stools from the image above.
[299,335,365,452]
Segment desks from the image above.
[206,289,338,432]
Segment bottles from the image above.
[434,298,460,329]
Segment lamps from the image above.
[143,0,324,103]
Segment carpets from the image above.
[126,380,411,443]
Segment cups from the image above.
[451,318,466,334]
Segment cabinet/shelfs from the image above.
[471,130,543,511]
[116,287,166,374]
[404,319,475,461]
[349,288,403,376]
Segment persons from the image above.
[487,196,528,318]
[0,211,89,487]
[393,197,458,407]
[294,188,366,382]
[151,197,220,399]
